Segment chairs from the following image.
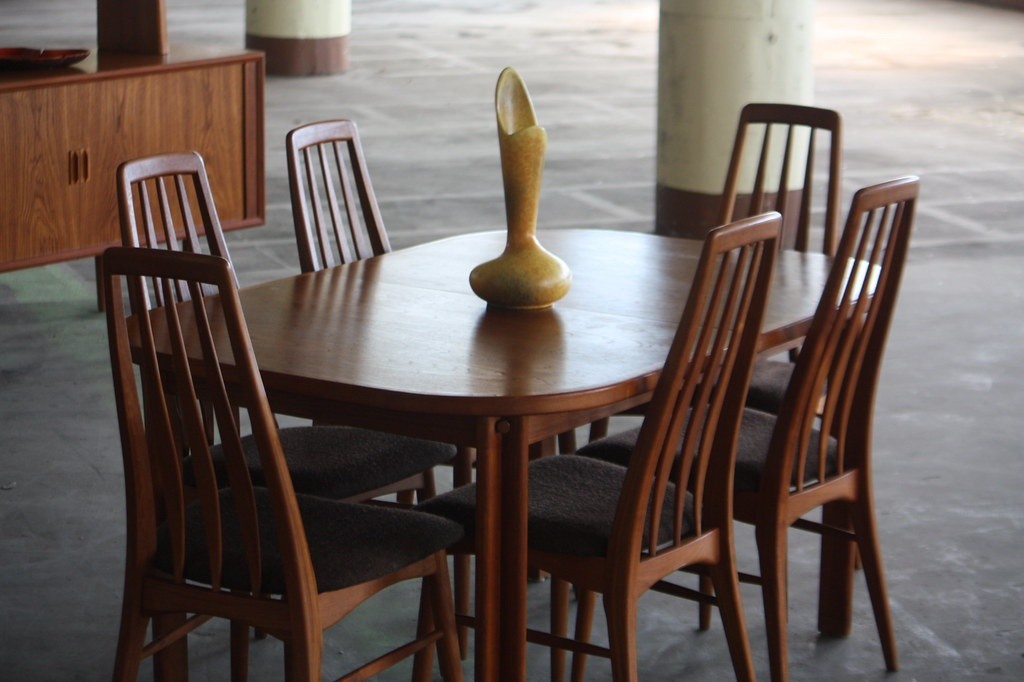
[589,102,840,444]
[411,210,786,682]
[99,243,468,682]
[550,173,920,681]
[117,151,467,660]
[286,117,577,504]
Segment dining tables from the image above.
[126,227,884,682]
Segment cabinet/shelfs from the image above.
[0,44,266,274]
